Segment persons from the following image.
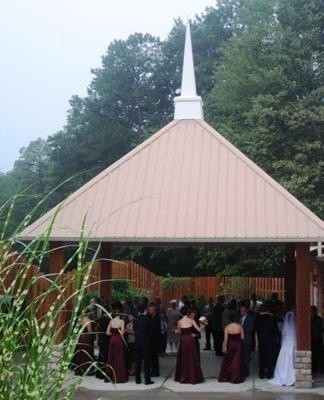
[132,305,160,384]
[269,309,296,386]
[105,309,129,383]
[68,292,324,379]
[218,312,249,383]
[173,307,204,384]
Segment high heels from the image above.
[128,367,132,373]
[130,368,136,376]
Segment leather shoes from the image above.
[215,351,225,357]
[203,347,212,351]
[145,380,154,385]
[149,372,161,377]
[135,380,142,384]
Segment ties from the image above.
[240,316,243,325]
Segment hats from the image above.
[169,298,178,304]
